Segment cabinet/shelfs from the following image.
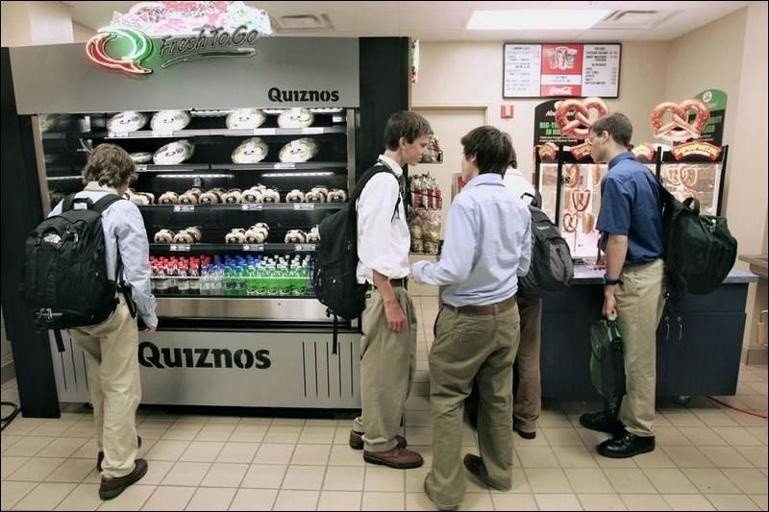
[0,36,412,418]
[538,142,729,260]
[654,311,746,397]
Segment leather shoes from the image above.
[464,454,482,478]
[349,430,407,451]
[98,459,147,500]
[513,426,535,439]
[597,433,655,457]
[363,445,424,470]
[97,436,142,471]
[579,412,624,435]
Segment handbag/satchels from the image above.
[589,317,626,398]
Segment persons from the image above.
[349,110,433,471]
[48,144,160,500]
[503,147,542,440]
[579,112,668,459]
[411,126,532,512]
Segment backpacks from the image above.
[519,186,574,291]
[312,164,402,355]
[20,193,126,352]
[646,164,738,300]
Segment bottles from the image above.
[148,254,315,296]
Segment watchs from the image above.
[602,274,624,286]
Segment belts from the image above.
[367,278,409,289]
[442,296,517,316]
